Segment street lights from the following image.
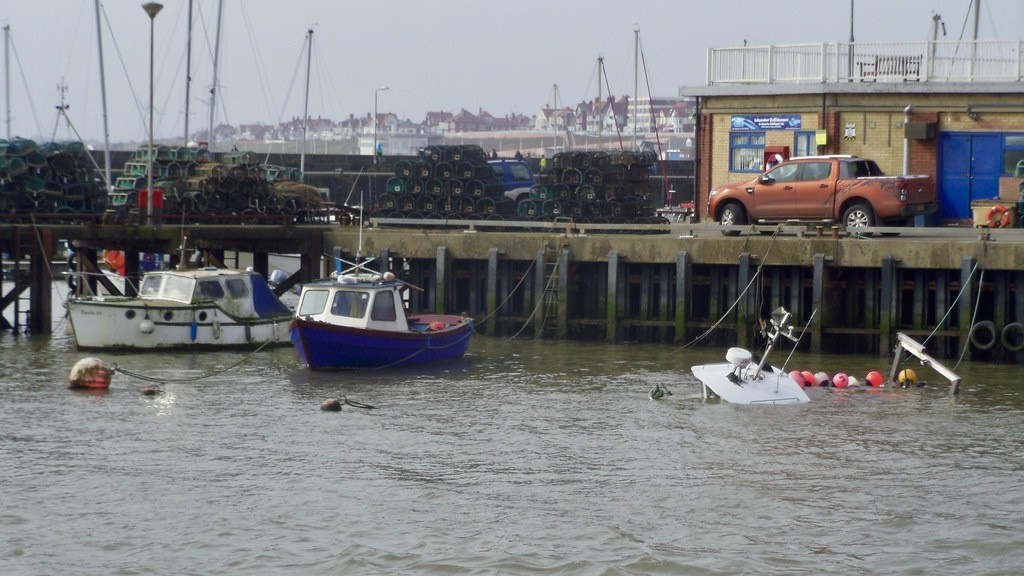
[139,2,165,224]
[372,86,391,204]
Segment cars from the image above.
[487,159,538,204]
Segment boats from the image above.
[286,192,475,370]
[60,263,299,352]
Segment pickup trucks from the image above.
[708,153,938,235]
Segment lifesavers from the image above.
[985,205,1010,229]
[999,322,1024,352]
[67,274,78,290]
[970,321,997,350]
[67,239,78,252]
[430,321,445,332]
[766,154,783,172]
[68,253,78,270]
